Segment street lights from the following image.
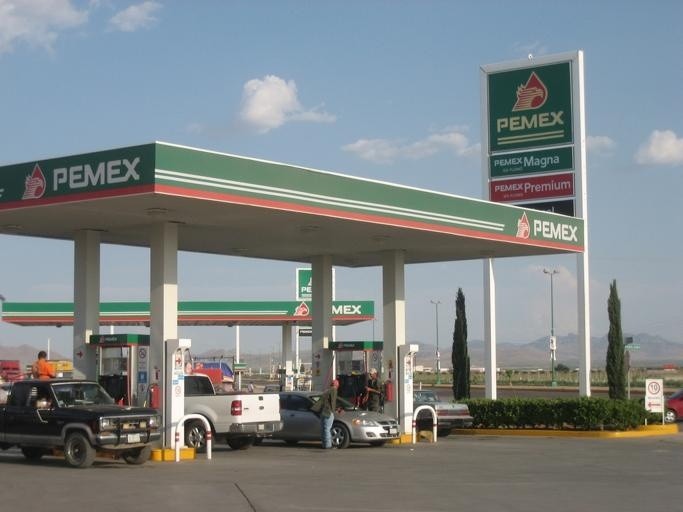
[430,300,443,385]
[543,268,560,387]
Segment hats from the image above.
[331,379,339,386]
[368,368,378,374]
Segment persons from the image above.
[248,381,254,393]
[361,367,382,413]
[32,350,56,380]
[310,380,346,451]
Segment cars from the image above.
[664,387,683,424]
[413,389,475,437]
[256,390,402,449]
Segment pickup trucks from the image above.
[0,377,163,469]
[184,370,284,453]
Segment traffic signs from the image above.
[644,378,664,413]
